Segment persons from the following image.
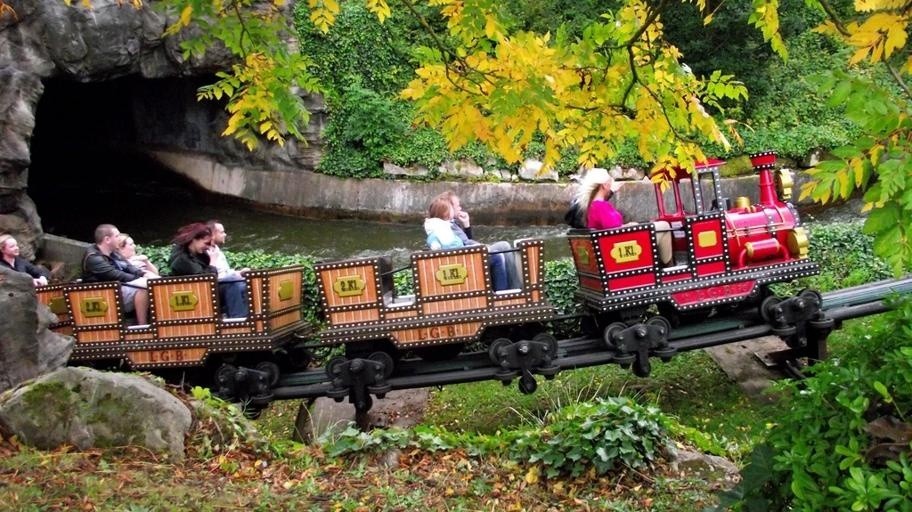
[564,180,618,228]
[168,223,248,319]
[444,190,520,289]
[202,220,250,292]
[0,233,48,288]
[115,234,160,276]
[571,167,685,268]
[423,195,509,290]
[82,224,162,325]
